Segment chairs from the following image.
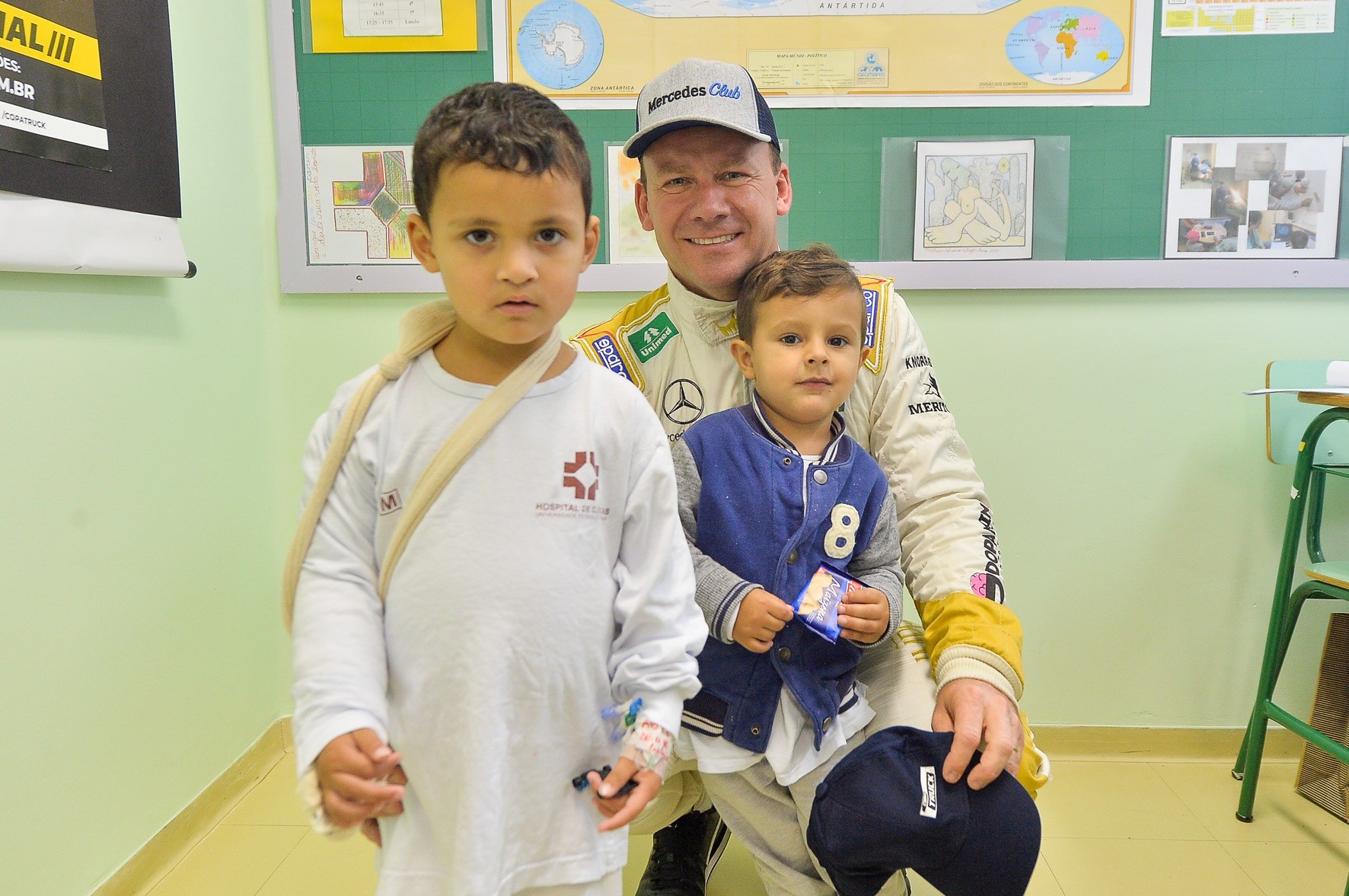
[1232,354,1349,779]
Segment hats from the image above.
[622,58,781,159]
[806,727,1041,896]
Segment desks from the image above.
[1234,392,1349,896]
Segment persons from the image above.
[1214,181,1228,217]
[1191,152,1207,181]
[562,57,1045,896]
[1178,218,1238,251]
[1247,210,1271,249]
[1268,171,1313,210]
[617,243,904,896]
[1289,230,1309,249]
[290,82,710,896]
